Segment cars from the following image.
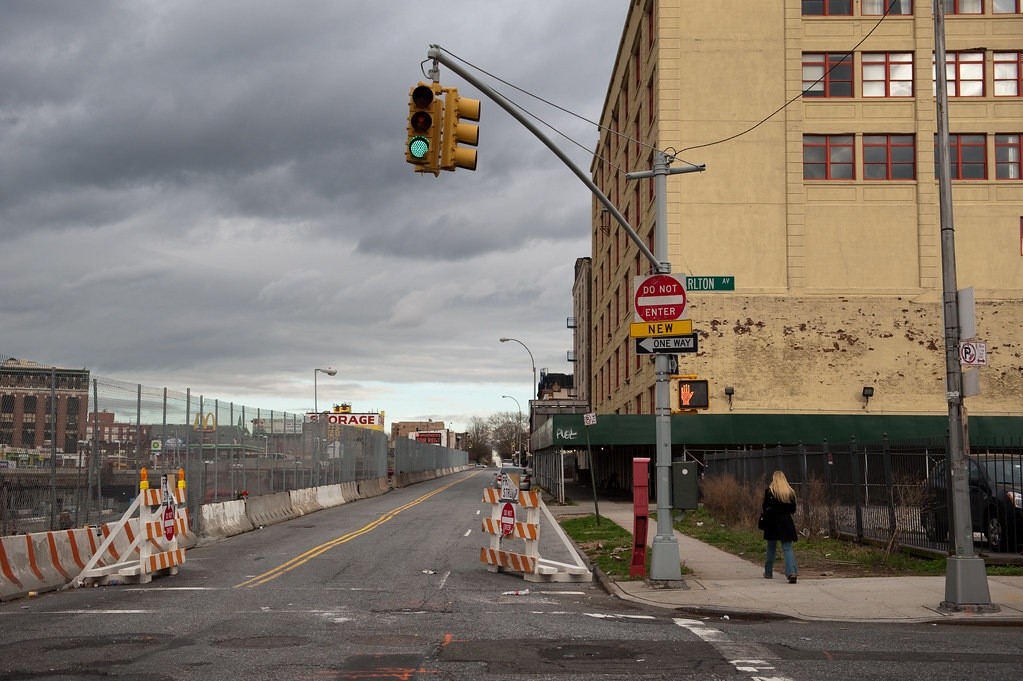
[494,467,530,490]
[476,463,487,468]
[920,454,1023,553]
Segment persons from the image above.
[761,471,797,584]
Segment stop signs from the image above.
[162,506,177,544]
[633,274,686,322]
[500,503,515,534]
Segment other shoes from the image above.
[788,573,799,584]
[762,571,772,578]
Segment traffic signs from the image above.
[633,332,698,354]
[687,277,735,291]
[630,321,695,338]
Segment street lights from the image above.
[502,395,522,467]
[314,366,337,492]
[499,337,536,478]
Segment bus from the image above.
[182,444,258,461]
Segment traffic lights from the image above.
[406,85,434,165]
[678,379,709,409]
[440,91,481,172]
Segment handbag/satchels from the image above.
[758,513,768,530]
